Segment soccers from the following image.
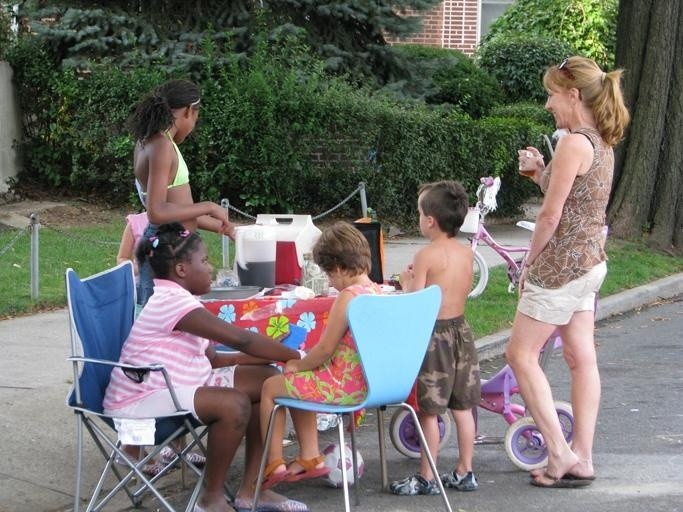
[320,442,365,488]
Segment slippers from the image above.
[530,461,595,488]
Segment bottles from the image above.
[315,411,340,433]
[302,252,329,296]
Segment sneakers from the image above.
[438,472,479,492]
[389,473,440,495]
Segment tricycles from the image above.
[387,295,598,472]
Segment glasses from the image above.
[558,58,582,101]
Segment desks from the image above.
[195,284,400,373]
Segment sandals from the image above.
[250,454,331,493]
[234,497,309,512]
[114,446,207,476]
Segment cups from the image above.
[214,267,235,287]
[517,149,540,178]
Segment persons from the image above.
[101,222,312,512]
[505,56,631,487]
[389,181,481,495]
[126,80,235,308]
[117,211,151,305]
[253,220,383,492]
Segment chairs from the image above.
[231,214,321,287]
[250,284,452,511]
[61,260,234,512]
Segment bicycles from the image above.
[459,177,607,300]
